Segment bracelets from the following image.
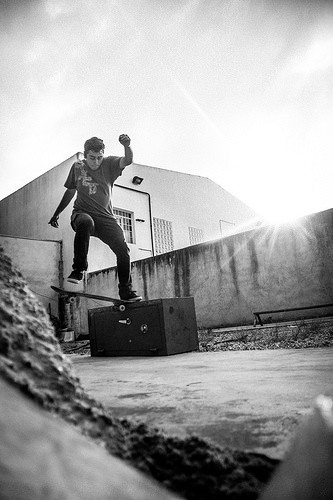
[52,215,59,222]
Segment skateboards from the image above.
[49,285,136,312]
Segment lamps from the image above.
[132,176,143,184]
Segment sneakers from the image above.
[120,290,142,300]
[66,271,83,282]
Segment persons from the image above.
[48,134,142,302]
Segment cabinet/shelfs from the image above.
[86,297,198,357]
